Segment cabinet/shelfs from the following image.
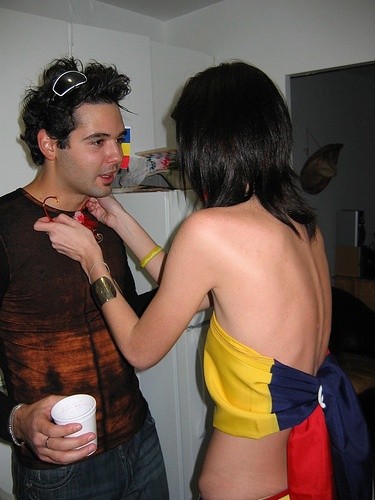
[334,244,375,312]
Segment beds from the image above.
[331,287,375,427]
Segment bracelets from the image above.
[9,403,28,447]
[89,262,123,307]
[139,247,164,268]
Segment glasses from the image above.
[51,70,87,101]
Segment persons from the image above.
[0,58,169,500]
[34,62,370,500]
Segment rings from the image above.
[45,437,50,448]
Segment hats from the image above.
[300,142,344,196]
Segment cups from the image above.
[51,394,98,457]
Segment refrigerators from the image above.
[102,191,236,498]
